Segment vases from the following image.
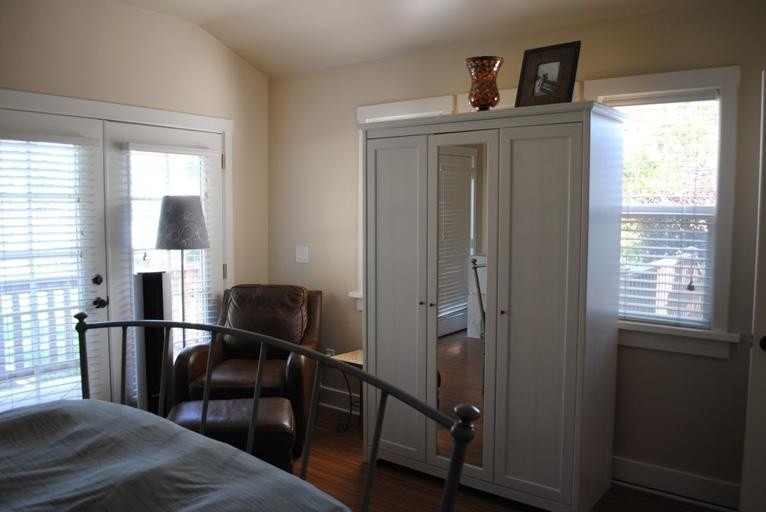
[464,56,504,111]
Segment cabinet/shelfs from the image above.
[357,100,624,511]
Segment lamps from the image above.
[154,194,211,353]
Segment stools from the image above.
[165,396,298,475]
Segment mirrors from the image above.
[428,140,489,472]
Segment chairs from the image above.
[172,283,324,459]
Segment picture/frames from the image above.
[513,38,582,108]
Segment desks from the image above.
[329,347,363,437]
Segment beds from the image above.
[0,313,481,512]
